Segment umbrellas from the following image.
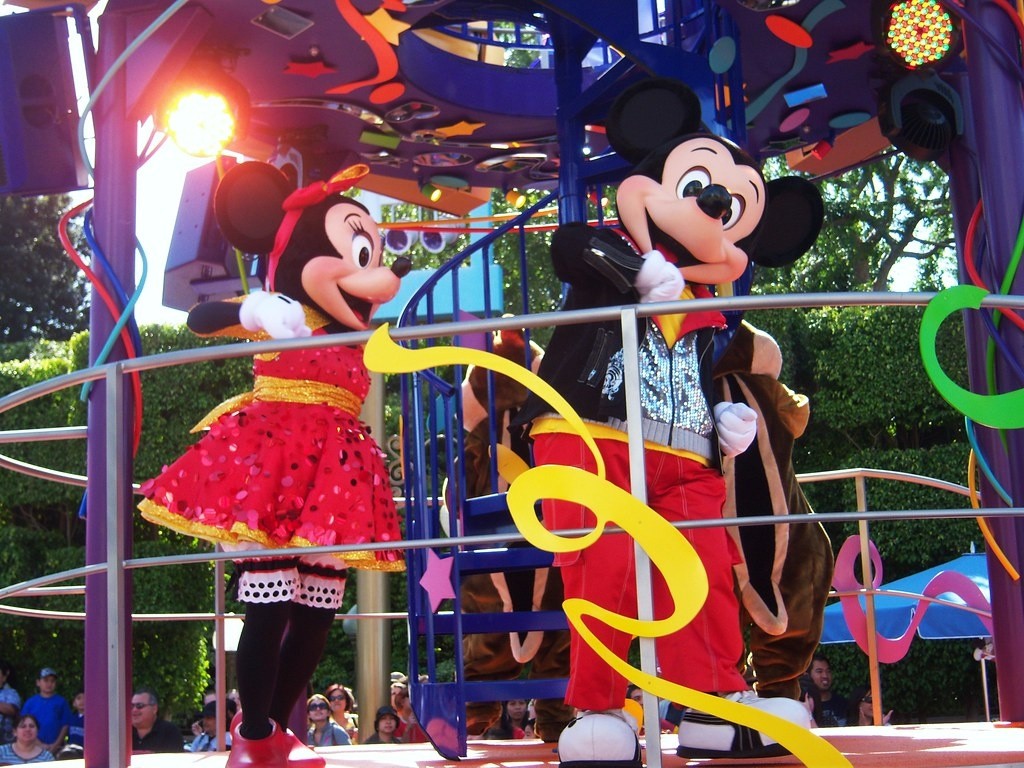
[817,541,992,722]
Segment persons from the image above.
[837,685,894,726]
[0,668,84,766]
[307,685,358,747]
[467,698,539,740]
[799,654,854,728]
[365,672,430,744]
[626,686,687,735]
[132,690,240,754]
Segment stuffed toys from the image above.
[507,76,824,768]
[442,336,577,743]
[711,319,834,699]
[135,161,410,768]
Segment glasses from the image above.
[862,697,872,703]
[132,702,152,709]
[307,703,327,711]
[329,694,344,702]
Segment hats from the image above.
[390,672,404,683]
[374,706,400,731]
[39,668,57,679]
